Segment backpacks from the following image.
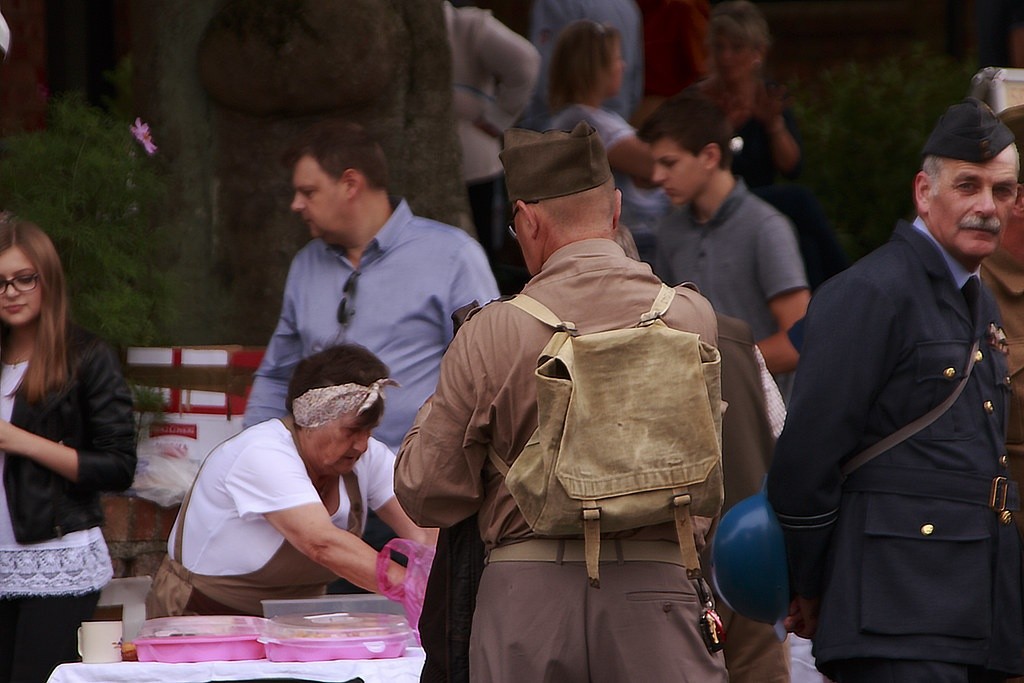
[465,281,723,588]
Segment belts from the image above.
[488,538,685,566]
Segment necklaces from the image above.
[1,338,36,370]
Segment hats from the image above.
[498,120,611,201]
[923,95,1016,161]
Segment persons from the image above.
[613,222,828,682]
[395,119,727,683]
[635,89,813,411]
[240,117,501,683]
[0,217,139,683]
[143,342,441,618]
[442,0,851,298]
[764,94,1023,682]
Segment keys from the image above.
[698,578,725,656]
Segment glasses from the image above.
[0,270,39,293]
[337,270,361,321]
